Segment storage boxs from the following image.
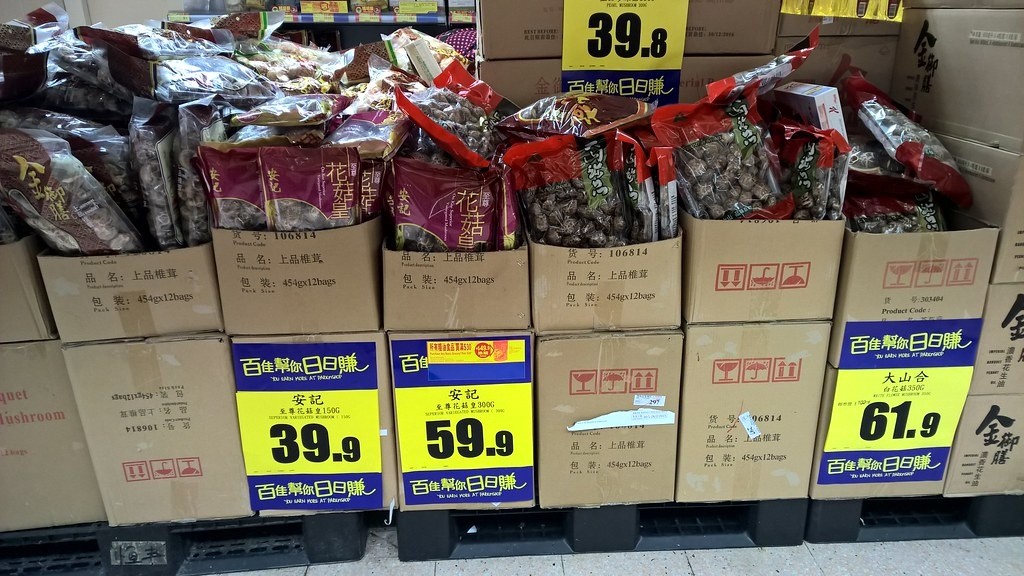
[473,0,1024,212]
[0,213,1024,535]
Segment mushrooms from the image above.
[0,23,973,253]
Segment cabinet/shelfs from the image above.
[166,0,479,49]
